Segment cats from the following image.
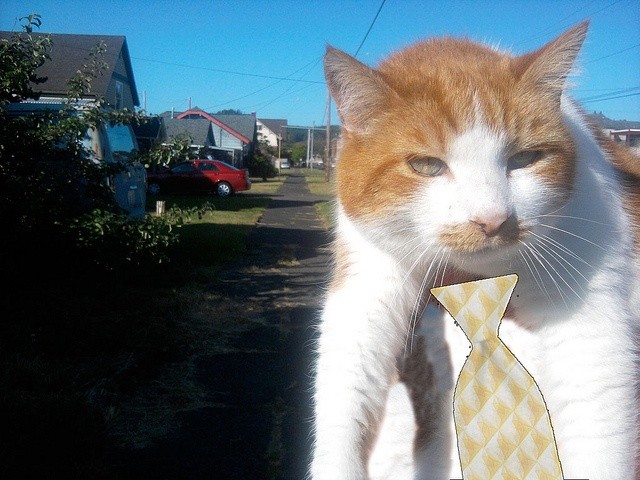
[309,18,639,479]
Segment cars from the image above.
[280,162,290,169]
[147,159,250,200]
